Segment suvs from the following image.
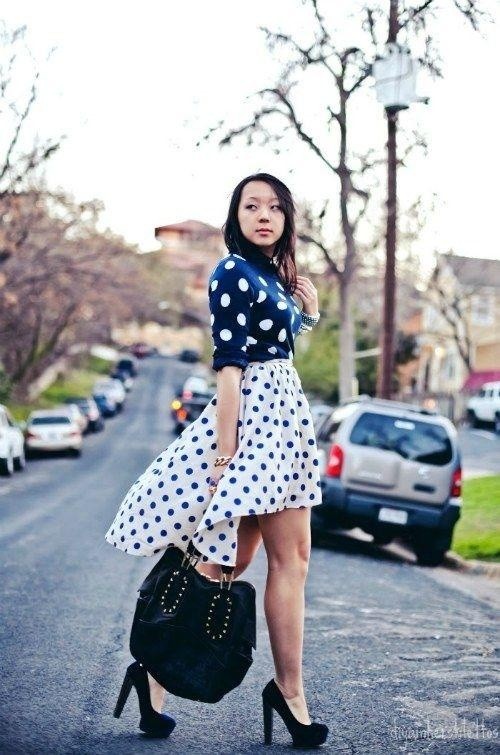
[0,402,29,476]
[311,391,467,568]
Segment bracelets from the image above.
[213,456,234,467]
[296,310,321,338]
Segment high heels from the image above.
[113,663,176,739]
[261,678,329,748]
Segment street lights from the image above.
[371,36,422,398]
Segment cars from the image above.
[22,339,216,459]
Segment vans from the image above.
[466,380,500,434]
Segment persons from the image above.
[105,170,339,750]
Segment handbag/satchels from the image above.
[130,546,256,703]
